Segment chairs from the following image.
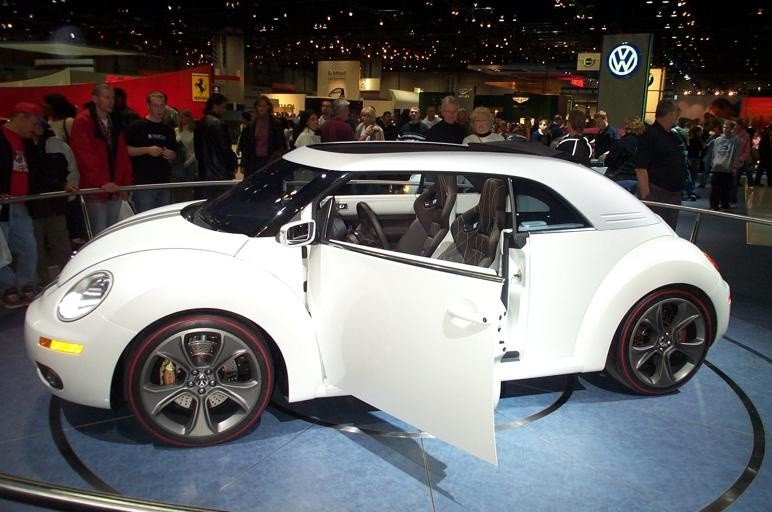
[437,177,509,268]
[393,172,457,257]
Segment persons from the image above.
[235,95,385,181]
[688,112,771,214]
[383,96,512,144]
[126,90,200,215]
[196,94,238,200]
[513,96,686,237]
[2,80,136,309]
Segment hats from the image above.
[14,103,50,128]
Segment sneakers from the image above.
[0,288,24,309]
[18,285,36,304]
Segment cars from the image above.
[23,137,736,470]
[559,127,626,151]
